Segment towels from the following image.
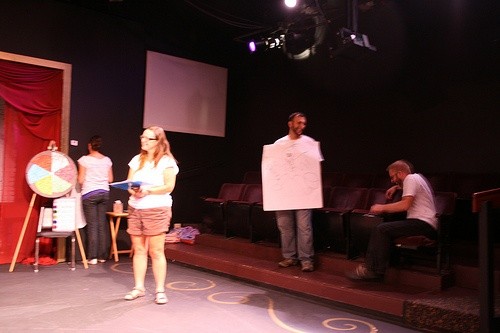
[167,226,200,240]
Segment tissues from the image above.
[113,199,124,214]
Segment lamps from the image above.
[247,35,280,55]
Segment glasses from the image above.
[139,135,157,141]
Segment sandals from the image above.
[155,291,168,304]
[124,287,145,299]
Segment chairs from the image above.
[33,207,75,271]
[200,172,497,276]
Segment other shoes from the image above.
[81,258,98,265]
[98,259,107,263]
[301,262,315,271]
[278,259,298,267]
[348,263,375,280]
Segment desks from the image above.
[106,211,134,262]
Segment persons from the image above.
[352,160,438,281]
[78,136,114,265]
[273,112,325,272]
[124,126,179,304]
